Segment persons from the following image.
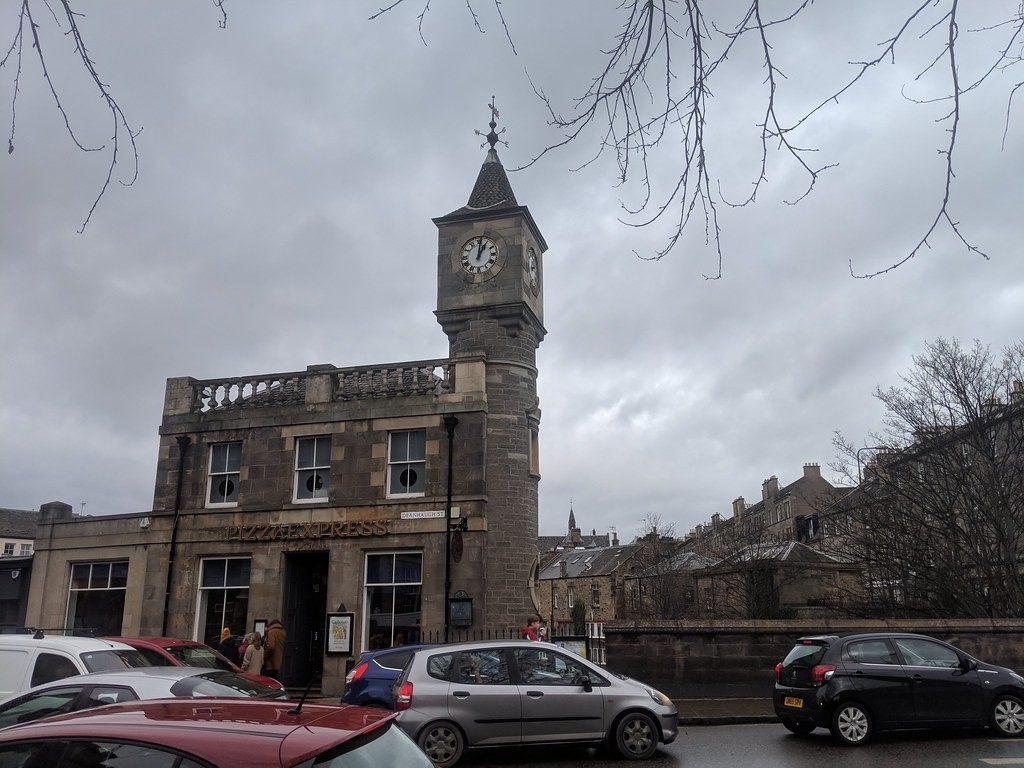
[263,619,286,682]
[237,633,255,659]
[240,632,264,677]
[216,628,240,674]
[522,614,541,643]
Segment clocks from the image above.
[451,228,508,284]
[528,247,540,298]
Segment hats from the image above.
[221,628,230,640]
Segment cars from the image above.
[0,698,442,768]
[773,630,1024,745]
[392,640,678,768]
[97,636,247,672]
[340,642,449,711]
[0,666,292,731]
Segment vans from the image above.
[0,627,153,706]
